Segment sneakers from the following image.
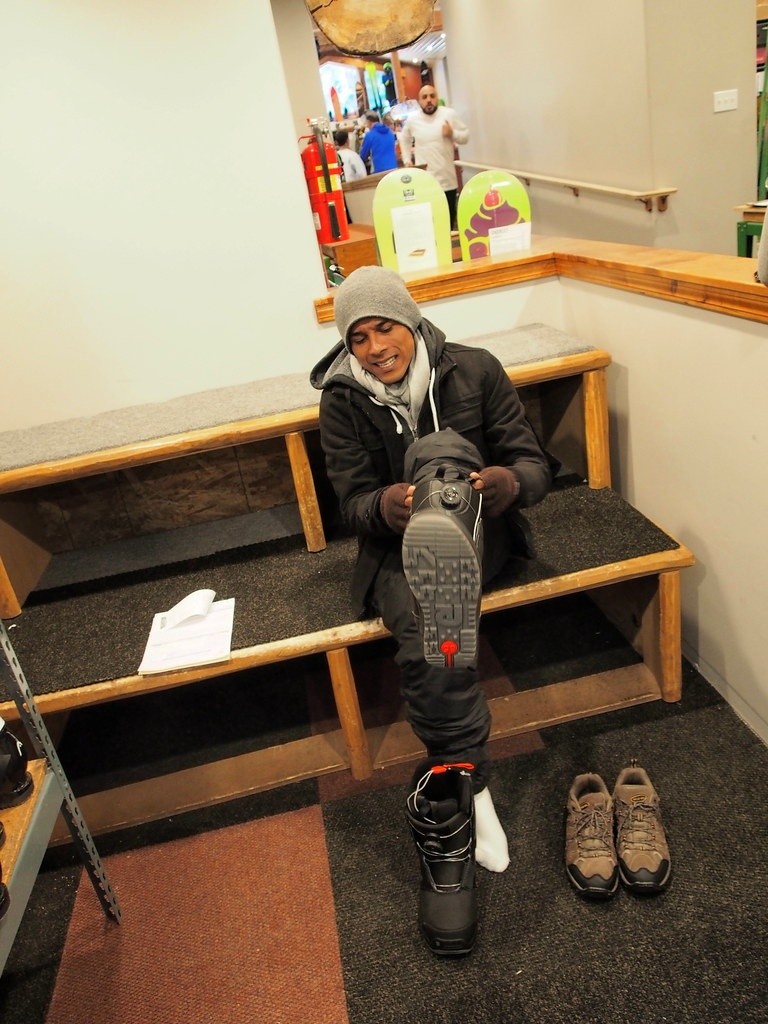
[611,759,670,890]
[565,772,619,896]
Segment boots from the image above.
[405,756,477,954]
[402,478,483,670]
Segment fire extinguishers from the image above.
[298,118,350,244]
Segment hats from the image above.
[332,265,422,355]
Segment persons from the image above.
[335,132,367,183]
[398,84,470,230]
[359,113,397,174]
[308,267,549,874]
[382,111,401,131]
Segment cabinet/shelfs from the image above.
[0,617,123,977]
[320,223,378,278]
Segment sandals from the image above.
[0,728,34,810]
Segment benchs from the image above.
[0,318,694,850]
